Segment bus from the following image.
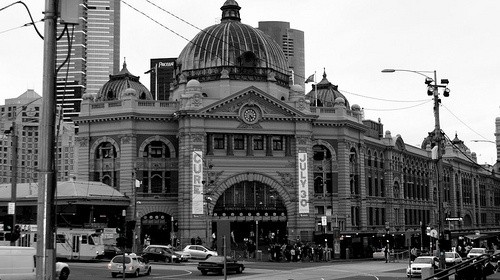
[0,223,105,261]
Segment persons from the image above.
[366,240,500,264]
[242,221,327,263]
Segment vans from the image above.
[0,244,36,280]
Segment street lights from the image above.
[381,68,445,269]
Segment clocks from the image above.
[243,107,259,125]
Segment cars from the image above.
[197,255,246,275]
[107,253,152,278]
[467,248,487,259]
[182,245,218,260]
[406,255,440,277]
[445,252,462,266]
[142,247,181,263]
[144,245,191,262]
[102,245,124,258]
